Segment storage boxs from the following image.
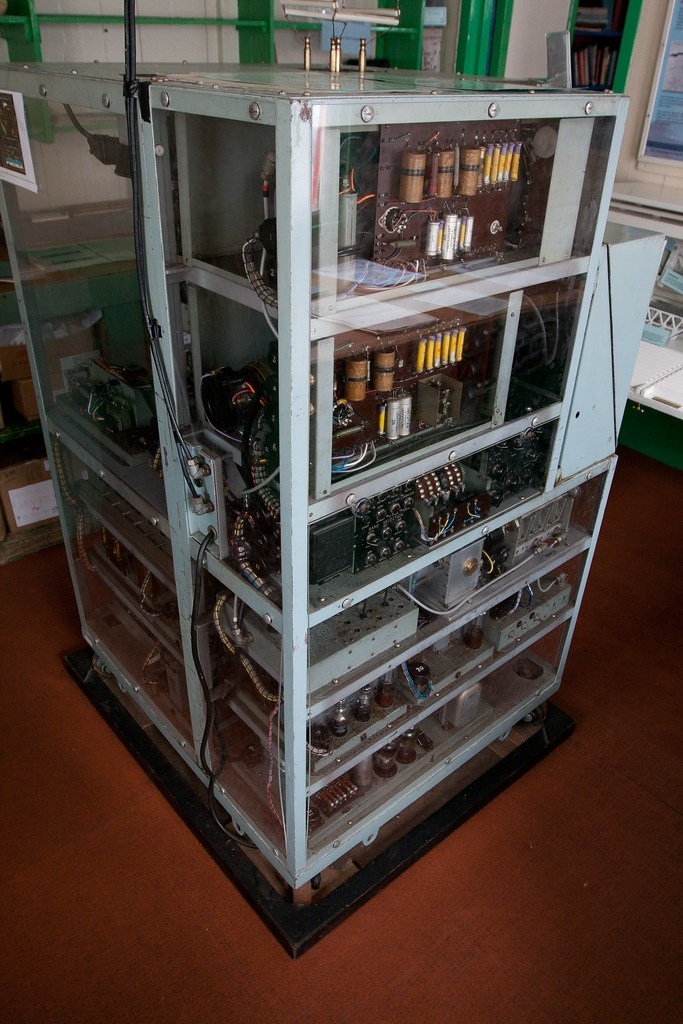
[11,371,63,422]
[0,449,88,536]
[0,313,95,383]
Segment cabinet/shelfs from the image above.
[0,1,674,887]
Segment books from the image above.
[574,45,617,87]
[574,7,610,32]
